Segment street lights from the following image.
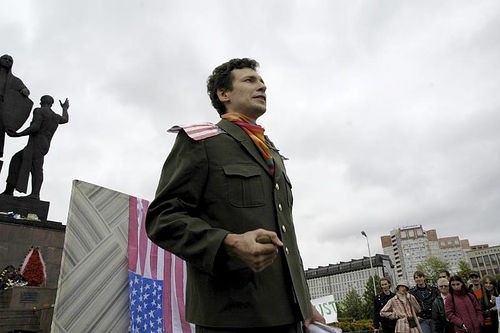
[361,230,377,296]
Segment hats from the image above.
[396,280,409,291]
[470,271,481,278]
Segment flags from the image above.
[127,195,195,333]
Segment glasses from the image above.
[439,276,446,278]
[451,283,460,286]
[439,285,448,288]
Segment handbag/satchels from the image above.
[407,316,418,328]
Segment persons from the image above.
[145,58,327,333]
[373,270,500,333]
[0,55,70,200]
